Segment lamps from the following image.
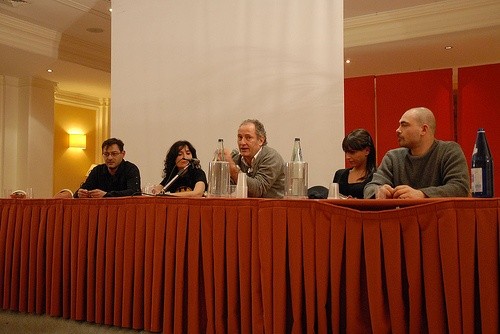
[69,134,87,149]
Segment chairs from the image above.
[56,189,74,198]
[308,186,329,199]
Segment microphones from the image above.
[231,148,240,158]
[183,157,200,164]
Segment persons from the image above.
[218,119,285,198]
[73,138,141,198]
[364,107,470,198]
[332,129,378,198]
[152,140,208,197]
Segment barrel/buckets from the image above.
[282,160,308,200]
[207,161,231,199]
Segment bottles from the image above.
[471,128,493,199]
[212,138,229,194]
[290,138,306,196]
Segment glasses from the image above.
[102,152,121,157]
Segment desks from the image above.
[0,197,500,334]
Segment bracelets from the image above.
[236,165,240,171]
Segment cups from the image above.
[327,183,339,199]
[235,173,248,198]
[25,187,32,199]
